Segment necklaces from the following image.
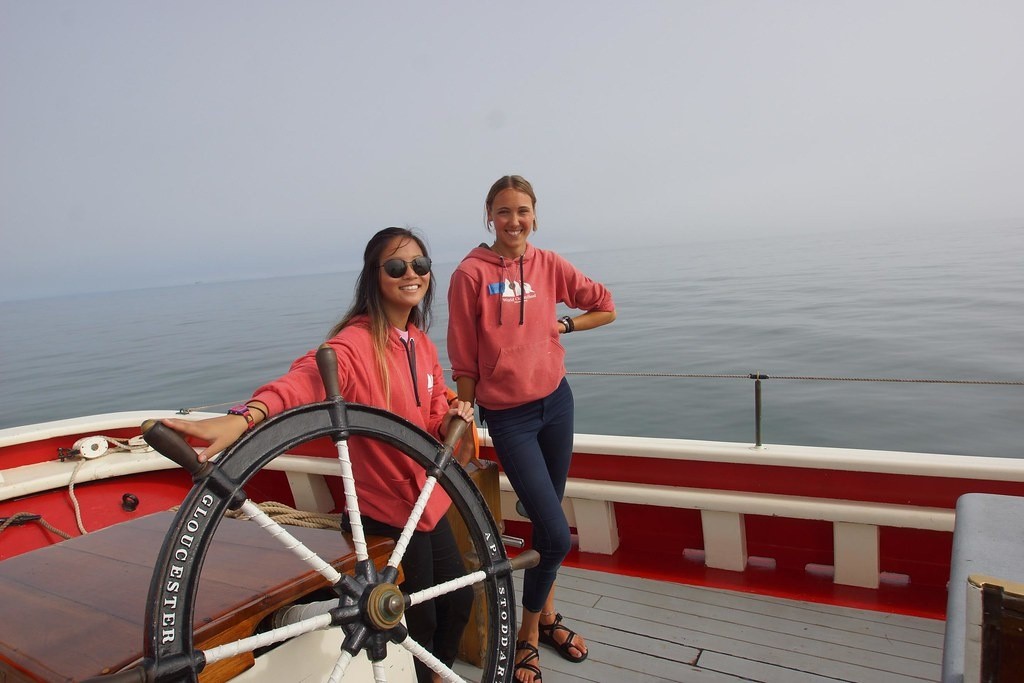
[494,241,521,289]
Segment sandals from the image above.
[539,613,589,663]
[514,639,542,683]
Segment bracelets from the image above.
[437,423,445,440]
[247,405,267,420]
[558,316,574,333]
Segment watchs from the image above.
[228,404,255,429]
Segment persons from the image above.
[446,175,617,683]
[162,227,475,683]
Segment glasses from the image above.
[376,256,432,278]
